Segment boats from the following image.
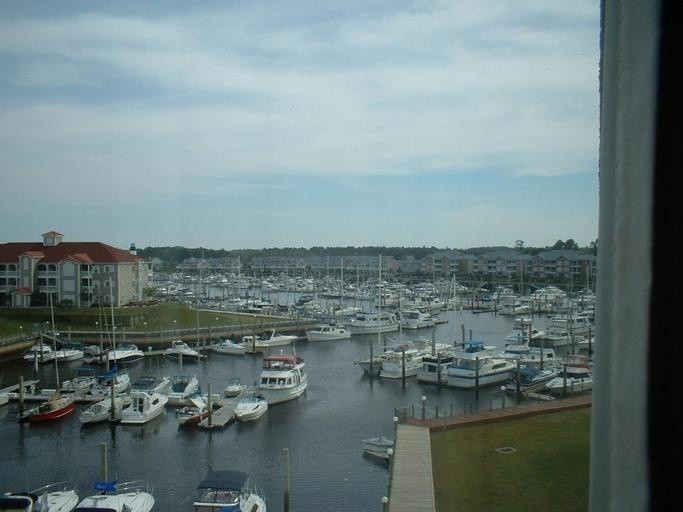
[74,478,154,511]
[0,474,81,512]
[191,469,266,512]
[18,312,307,429]
[362,435,394,461]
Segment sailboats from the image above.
[145,252,306,310]
